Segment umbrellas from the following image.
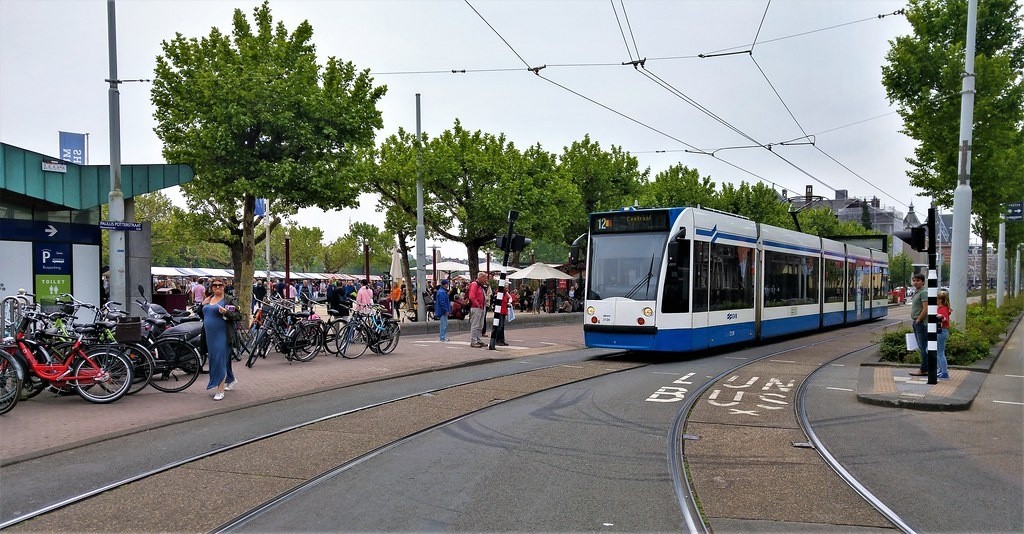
[504,262,574,304]
[408,260,564,291]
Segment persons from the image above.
[354,279,373,339]
[936,291,952,379]
[156,275,584,321]
[101,275,110,308]
[434,280,452,343]
[496,282,511,346]
[197,278,245,401]
[482,276,492,337]
[908,273,929,376]
[468,272,488,347]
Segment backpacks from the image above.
[462,281,481,310]
[455,301,468,315]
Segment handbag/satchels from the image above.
[371,299,374,305]
[906,333,919,351]
[508,305,515,322]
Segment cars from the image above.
[967,280,996,292]
[893,286,916,297]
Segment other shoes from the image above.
[936,375,949,380]
[225,378,238,391]
[440,337,450,342]
[496,341,508,346]
[482,334,488,337]
[213,390,225,400]
[909,371,928,376]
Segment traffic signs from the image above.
[1004,203,1022,220]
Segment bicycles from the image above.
[0,284,436,415]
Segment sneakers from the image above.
[470,342,482,347]
[477,342,488,346]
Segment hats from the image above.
[441,280,448,285]
[505,279,511,284]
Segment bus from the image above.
[569,195,891,352]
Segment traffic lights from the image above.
[508,210,520,222]
[893,227,926,253]
[510,234,525,251]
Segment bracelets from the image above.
[916,320,920,325]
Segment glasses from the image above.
[212,283,225,289]
